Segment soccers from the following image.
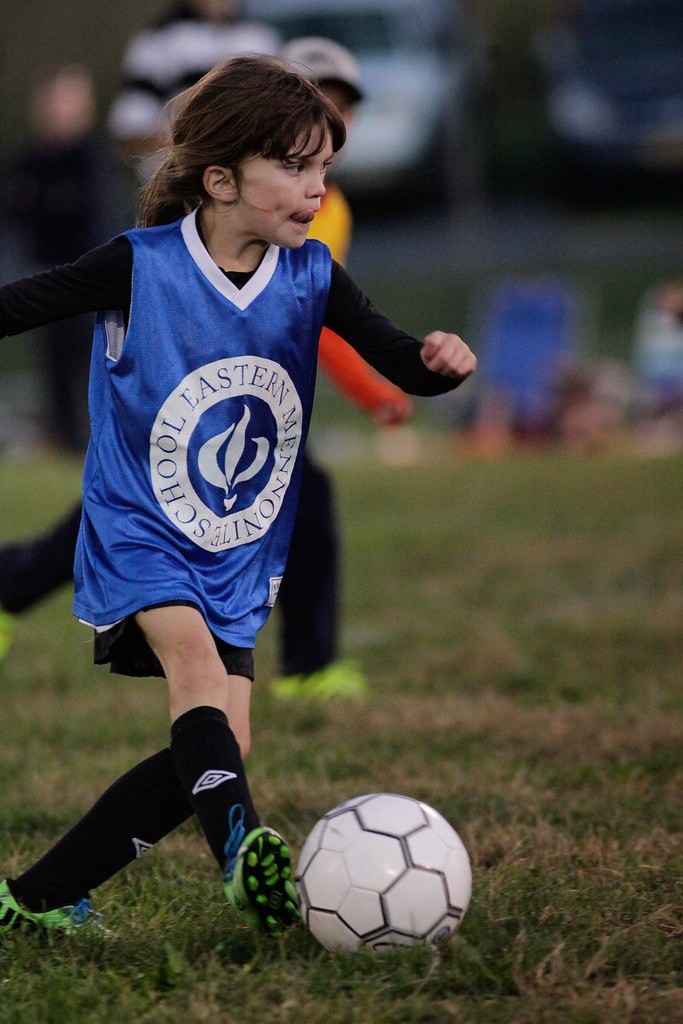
[293,796,472,951]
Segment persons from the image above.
[0,55,477,940]
[460,279,631,453]
[0,38,414,701]
[634,285,683,416]
[109,0,279,160]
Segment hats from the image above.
[284,36,367,115]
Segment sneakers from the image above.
[220,825,303,945]
[273,665,367,709]
[0,879,123,952]
